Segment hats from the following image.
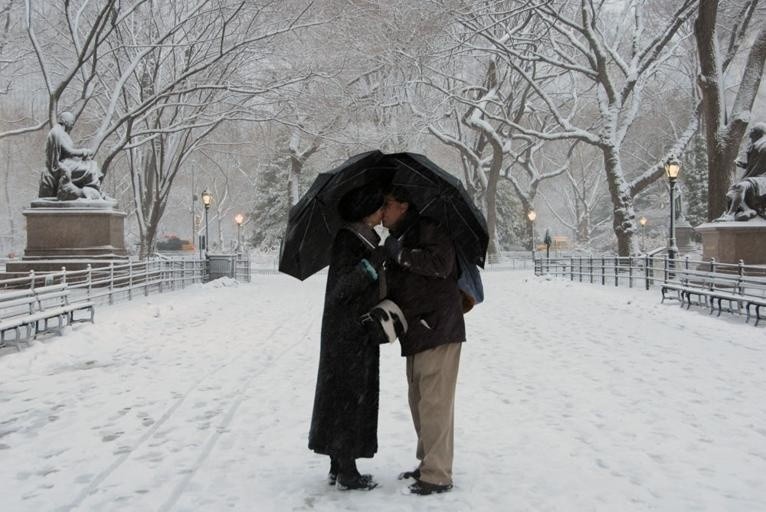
[337,184,383,222]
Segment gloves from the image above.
[383,237,402,257]
[368,246,384,269]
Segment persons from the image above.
[375,184,466,498]
[304,182,387,491]
[720,123,765,220]
[44,110,106,202]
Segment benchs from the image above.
[660,269,766,327]
[0,283,96,352]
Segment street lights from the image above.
[202,187,213,282]
[528,209,537,263]
[640,216,647,255]
[195,215,200,248]
[664,154,681,279]
[235,212,243,264]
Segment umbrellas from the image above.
[367,149,490,272]
[275,148,387,284]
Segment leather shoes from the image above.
[398,469,420,480]
[327,472,372,489]
[401,480,451,496]
